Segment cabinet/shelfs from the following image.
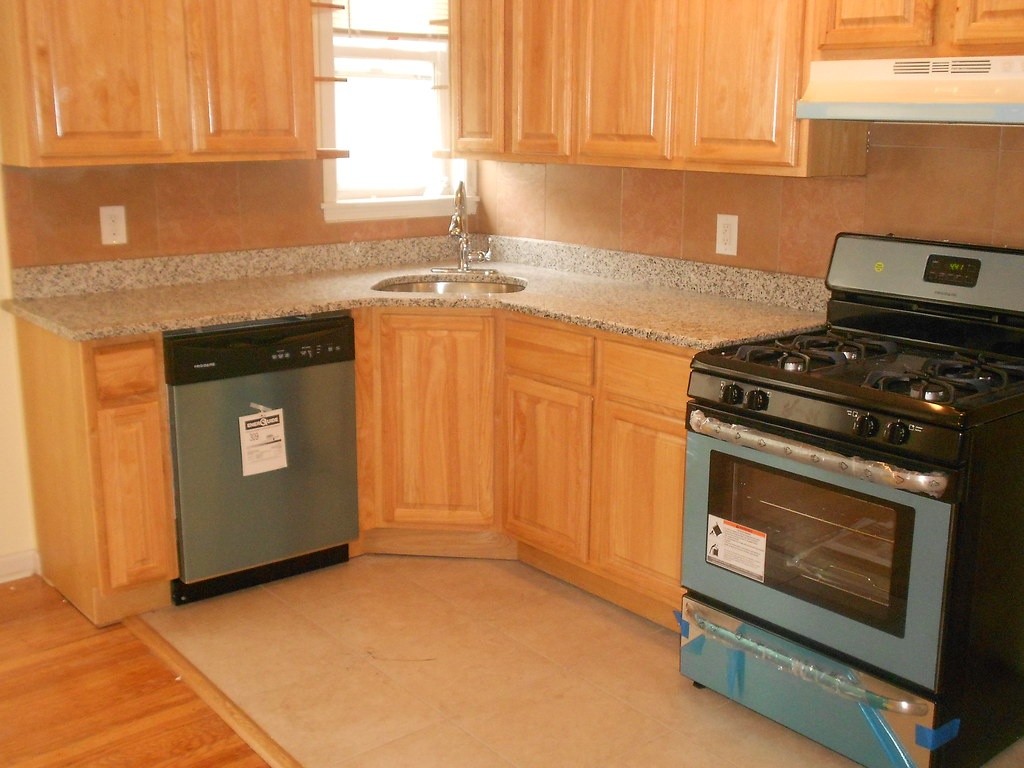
[811,0,1024,57]
[15,318,182,632]
[434,0,579,165]
[578,0,867,178]
[0,0,350,168]
[496,308,701,633]
[372,307,502,557]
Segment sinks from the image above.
[370,274,528,293]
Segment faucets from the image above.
[447,181,498,273]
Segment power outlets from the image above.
[717,214,739,256]
[100,205,126,245]
